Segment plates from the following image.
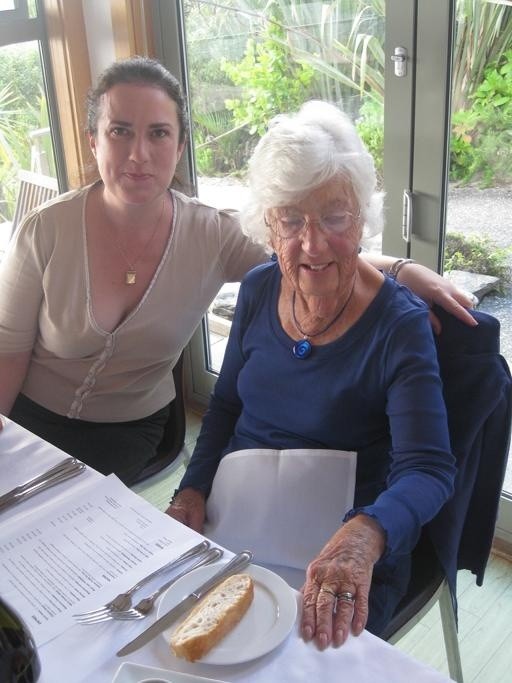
[157,562,298,666]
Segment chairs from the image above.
[380,306,512,683]
[131,349,190,493]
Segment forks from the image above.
[73,540,211,624]
[108,548,223,620]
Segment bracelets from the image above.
[383,252,418,291]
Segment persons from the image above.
[159,100,459,649]
[0,56,481,488]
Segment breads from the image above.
[170,573,252,663]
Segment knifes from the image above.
[2,454,87,514]
[114,550,253,655]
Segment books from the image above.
[0,468,210,655]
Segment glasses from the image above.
[264,206,363,240]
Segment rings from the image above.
[319,585,339,599]
[338,590,355,602]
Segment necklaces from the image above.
[290,271,360,359]
[95,182,171,288]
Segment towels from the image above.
[204,448,357,591]
